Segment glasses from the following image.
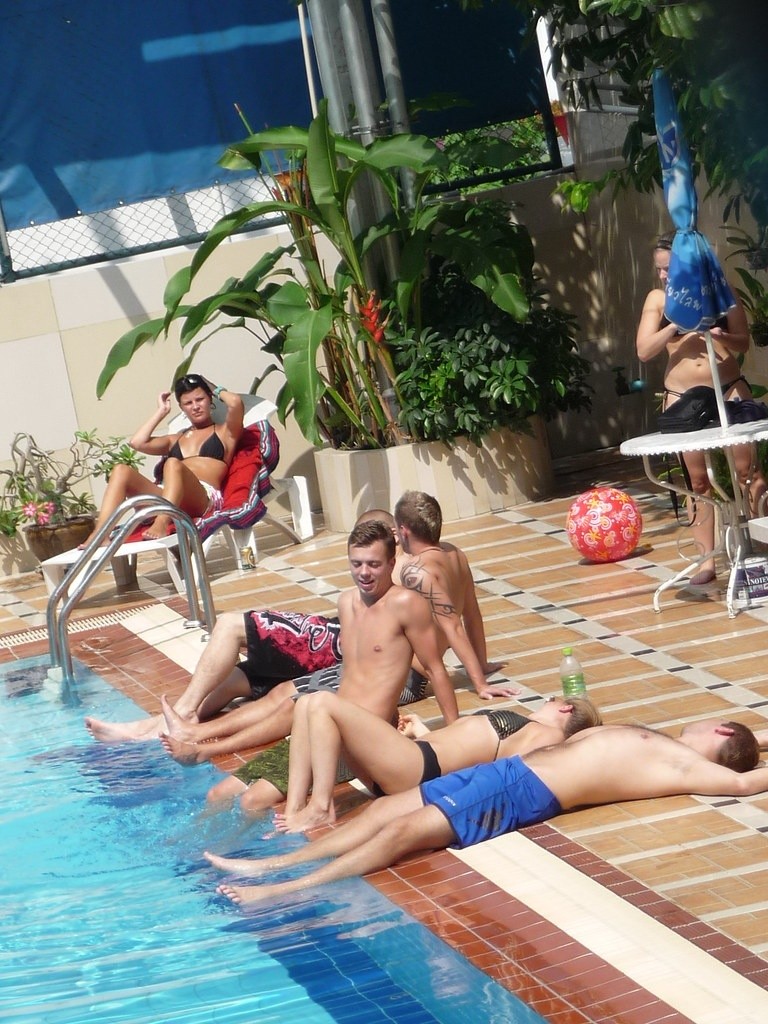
[175,377,211,396]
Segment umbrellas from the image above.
[647,60,744,524]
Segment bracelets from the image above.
[212,386,227,403]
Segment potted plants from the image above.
[95,94,598,534]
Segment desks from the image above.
[619,417,768,618]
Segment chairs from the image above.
[42,392,315,616]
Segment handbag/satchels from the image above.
[656,385,717,434]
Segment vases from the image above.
[22,517,95,561]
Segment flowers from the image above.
[0,427,147,537]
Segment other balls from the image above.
[568,486,641,562]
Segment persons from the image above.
[207,521,459,812]
[272,690,603,834]
[634,229,764,584]
[83,509,412,740]
[204,718,768,904]
[77,374,244,551]
[158,490,522,767]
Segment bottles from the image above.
[559,646,587,697]
